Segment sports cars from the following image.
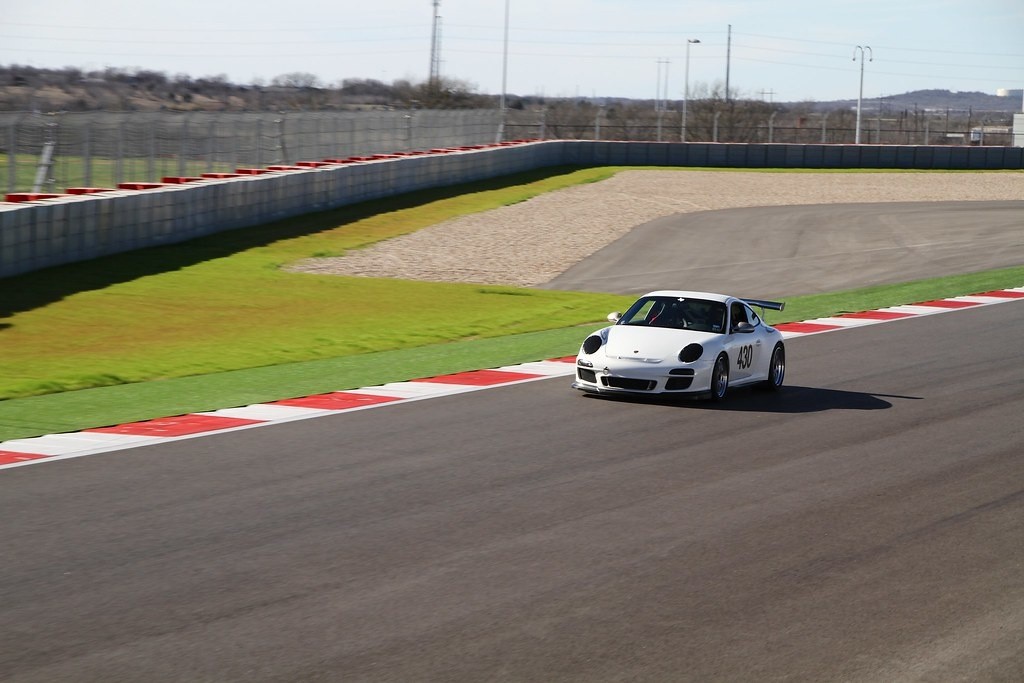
[571,292,788,398]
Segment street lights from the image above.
[678,38,701,142]
[851,43,873,144]
